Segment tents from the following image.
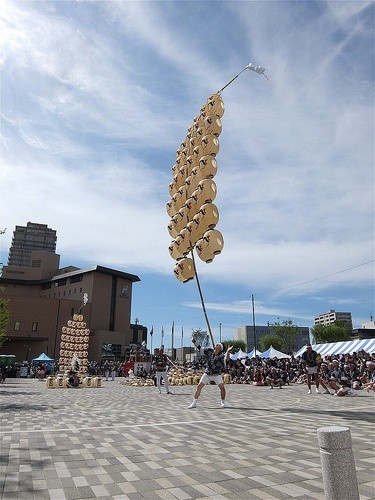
[293,339,375,361]
[230,345,291,360]
[32,352,55,364]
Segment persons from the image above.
[153,347,172,394]
[0,361,58,383]
[301,344,321,394]
[188,336,233,409]
[319,358,356,397]
[88,360,123,381]
[362,360,375,393]
[66,370,80,388]
[227,351,375,390]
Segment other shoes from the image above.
[158,390,162,394]
[323,391,330,394]
[188,403,197,409]
[221,402,227,408]
[316,390,321,394]
[308,391,312,394]
[167,392,173,395]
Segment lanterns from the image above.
[166,365,202,386]
[46,314,101,389]
[165,94,225,283]
[223,373,231,384]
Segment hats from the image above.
[306,343,312,349]
[158,347,164,352]
[217,342,225,352]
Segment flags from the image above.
[149,326,153,335]
